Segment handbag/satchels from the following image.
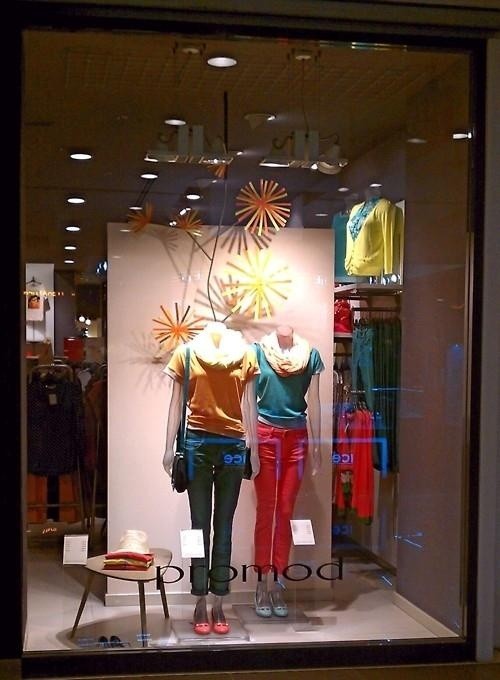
[171,454,187,493]
[244,447,252,480]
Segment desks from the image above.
[69,547,176,648]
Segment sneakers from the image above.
[111,636,124,648]
[98,635,109,649]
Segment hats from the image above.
[111,530,154,555]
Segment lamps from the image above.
[258,47,348,177]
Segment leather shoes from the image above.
[194,608,210,634]
[269,593,288,616]
[211,607,229,633]
[255,596,272,617]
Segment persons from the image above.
[160,321,262,637]
[341,184,399,281]
[244,324,326,618]
